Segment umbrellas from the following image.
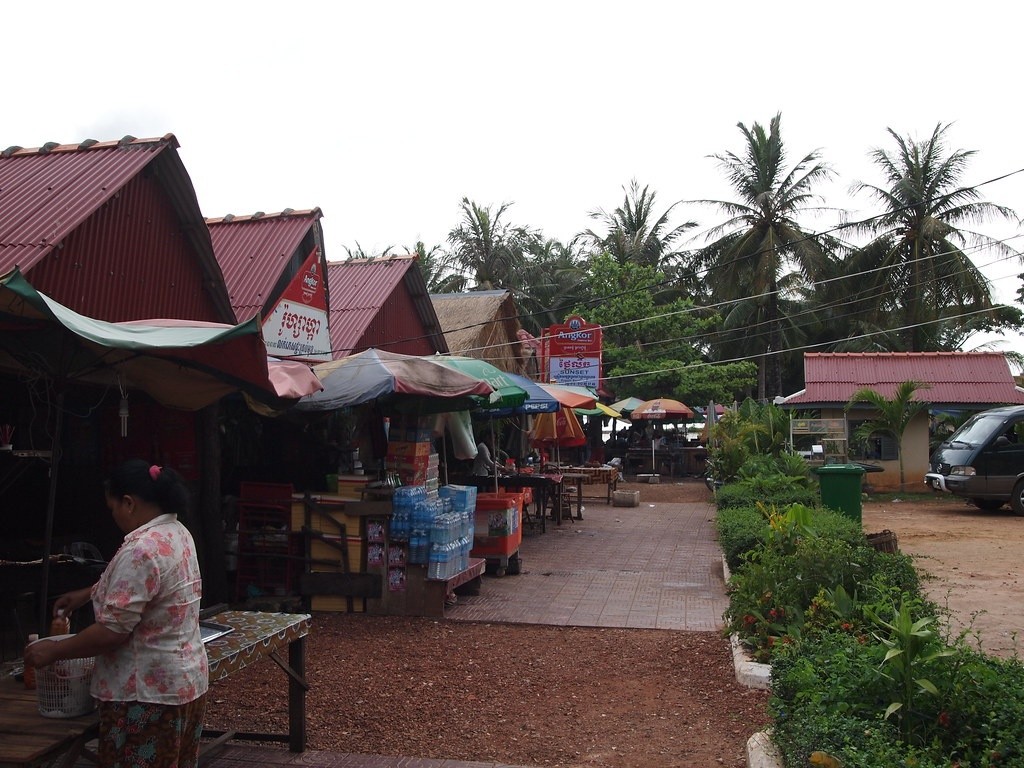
[610,395,763,470]
[0,264,610,634]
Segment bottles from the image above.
[385,472,473,580]
[23,634,41,690]
[49,608,70,637]
[533,448,540,476]
[505,459,515,471]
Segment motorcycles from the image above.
[702,455,739,491]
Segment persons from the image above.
[470,428,504,492]
[24,460,210,768]
[603,425,694,476]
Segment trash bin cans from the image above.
[810,463,868,530]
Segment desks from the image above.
[0,608,312,768]
[449,448,689,534]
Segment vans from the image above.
[924,405,1024,516]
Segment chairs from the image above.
[539,463,575,527]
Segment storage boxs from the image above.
[613,489,640,507]
[383,428,433,487]
[290,475,369,611]
[474,494,524,554]
